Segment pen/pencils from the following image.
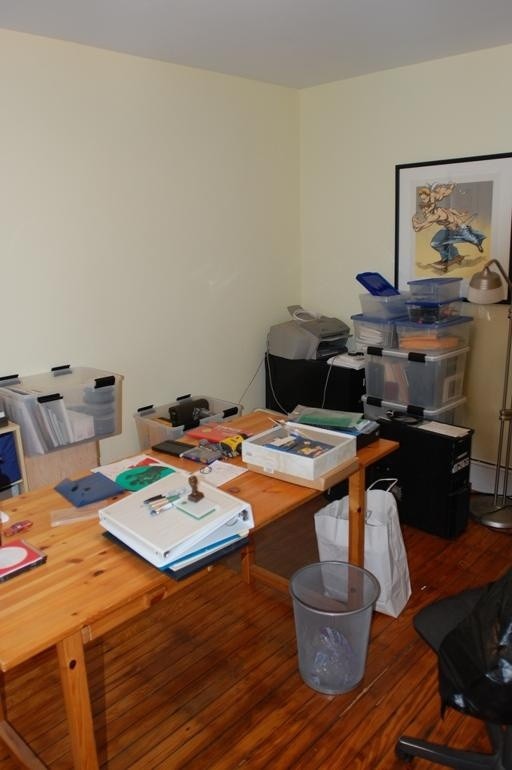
[143,493,188,513]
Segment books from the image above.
[0,416,380,585]
[358,324,391,346]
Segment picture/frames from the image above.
[390,150,511,307]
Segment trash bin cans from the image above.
[288,560,381,694]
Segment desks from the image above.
[0,408,406,770]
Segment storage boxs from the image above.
[1,363,124,458]
[345,265,476,428]
[132,392,243,456]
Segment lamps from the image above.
[467,257,512,538]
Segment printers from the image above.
[267,306,349,360]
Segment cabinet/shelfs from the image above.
[0,418,29,506]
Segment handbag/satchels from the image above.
[314,490,412,618]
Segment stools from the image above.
[391,578,512,770]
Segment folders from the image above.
[95,469,256,570]
